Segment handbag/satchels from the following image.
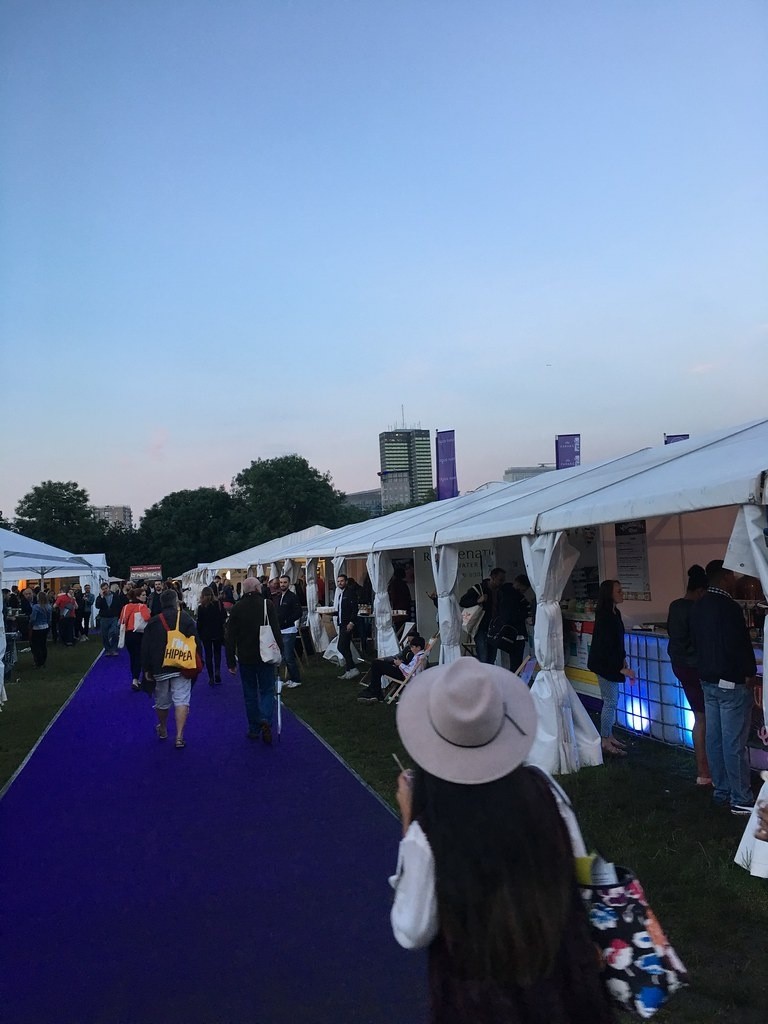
[486,616,518,646]
[181,655,202,677]
[118,604,128,649]
[162,611,197,670]
[259,599,282,667]
[461,583,487,638]
[525,764,690,1019]
[60,603,73,618]
[133,603,149,633]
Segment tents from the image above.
[2,554,110,629]
[248,420,768,776]
[172,525,330,611]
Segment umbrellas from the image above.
[0,528,110,591]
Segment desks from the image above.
[612,625,767,771]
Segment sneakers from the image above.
[730,798,756,814]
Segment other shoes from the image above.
[283,679,301,688]
[131,680,142,691]
[337,668,360,679]
[105,652,111,655]
[697,776,715,788]
[112,651,119,656]
[247,719,272,744]
[611,740,628,750]
[356,697,378,705]
[209,677,221,687]
[602,744,627,758]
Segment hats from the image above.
[395,656,538,785]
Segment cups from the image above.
[629,671,635,685]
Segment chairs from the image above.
[461,636,476,657]
[357,619,442,705]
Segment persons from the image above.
[430,591,439,628]
[459,567,534,673]
[387,569,411,635]
[141,590,201,747]
[257,575,314,689]
[2,583,95,678]
[225,576,284,743]
[95,577,187,692]
[325,574,363,679]
[357,632,427,703]
[588,580,634,755]
[667,564,711,785]
[390,656,613,1024]
[197,575,242,686]
[688,559,758,813]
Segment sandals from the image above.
[176,738,185,748]
[156,724,169,741]
